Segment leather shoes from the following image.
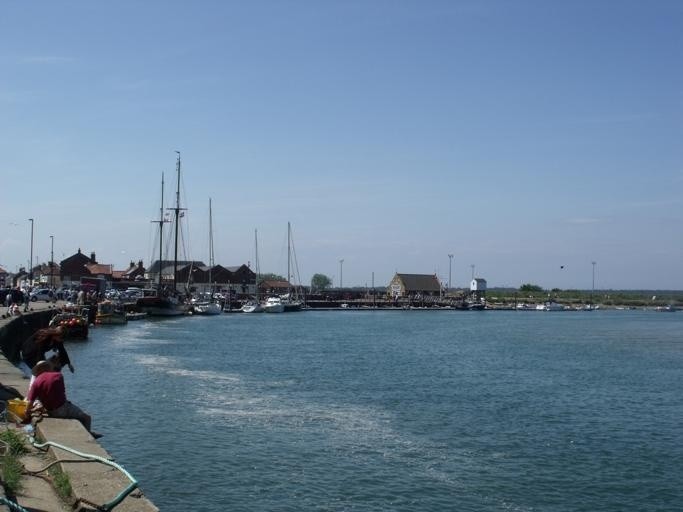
[92,433,103,439]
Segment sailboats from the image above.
[136,150,304,315]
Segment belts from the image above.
[55,402,65,408]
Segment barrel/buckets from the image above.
[6,400,28,423]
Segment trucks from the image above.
[80,276,105,300]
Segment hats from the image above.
[31,360,49,376]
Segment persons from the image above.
[25,360,104,439]
[19,324,75,374]
[0,287,105,316]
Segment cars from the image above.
[105,287,139,302]
[30,288,58,302]
[0,289,25,306]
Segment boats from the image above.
[536,302,564,311]
[95,301,127,324]
[656,305,675,312]
[48,312,89,339]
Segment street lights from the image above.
[592,261,596,290]
[28,218,34,287]
[448,254,454,289]
[50,236,54,288]
[339,259,344,288]
